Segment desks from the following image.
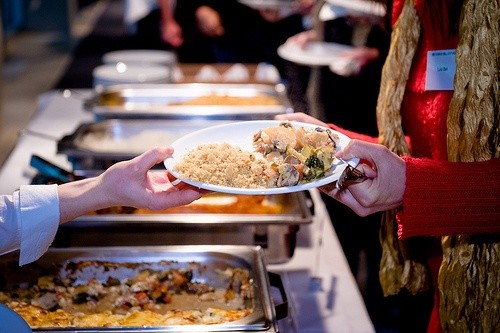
[0,89,376,333]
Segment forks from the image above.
[336,165,367,191]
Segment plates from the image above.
[325,0,387,18]
[91,50,176,92]
[239,0,300,11]
[277,41,353,66]
[163,120,360,194]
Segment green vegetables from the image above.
[304,155,325,181]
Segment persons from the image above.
[47,0,500,333]
[0,145,206,268]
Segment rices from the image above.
[174,141,272,189]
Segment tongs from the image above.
[31,155,138,214]
